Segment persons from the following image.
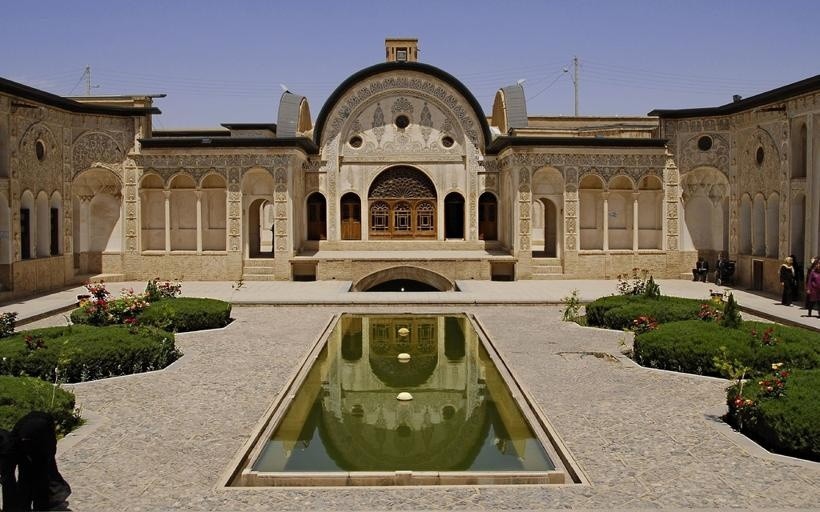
[692,255,709,283]
[806,255,819,309]
[778,257,796,307]
[806,260,820,317]
[714,251,725,284]
